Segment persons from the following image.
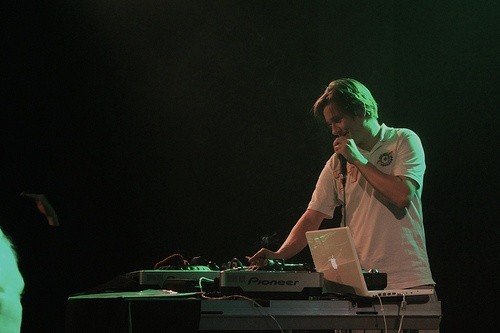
[248,77,442,333]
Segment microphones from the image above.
[338,154,347,189]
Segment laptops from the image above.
[305,225,434,298]
